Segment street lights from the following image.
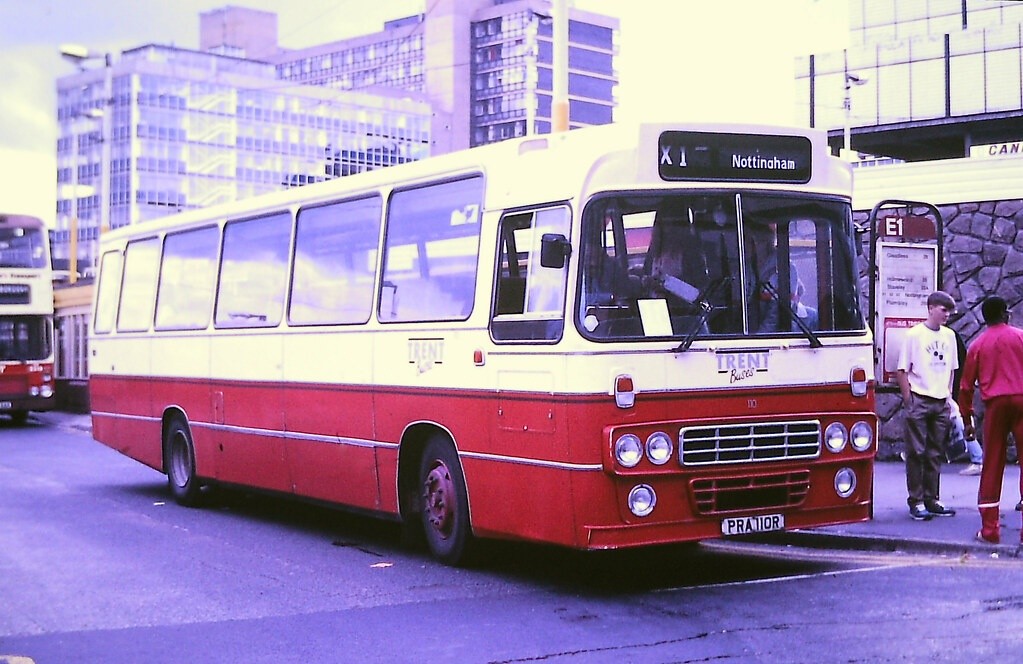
[844,48,869,163]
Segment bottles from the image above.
[966,436,983,462]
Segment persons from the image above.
[895,290,959,519]
[901,328,986,476]
[958,296,1023,545]
[548,220,822,338]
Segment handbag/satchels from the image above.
[944,398,968,463]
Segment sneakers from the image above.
[927,500,956,517]
[909,502,932,521]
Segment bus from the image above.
[0,213,56,425]
[86,123,879,569]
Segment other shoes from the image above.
[976,531,987,543]
[901,451,905,461]
[1015,501,1023,510]
[960,463,982,476]
